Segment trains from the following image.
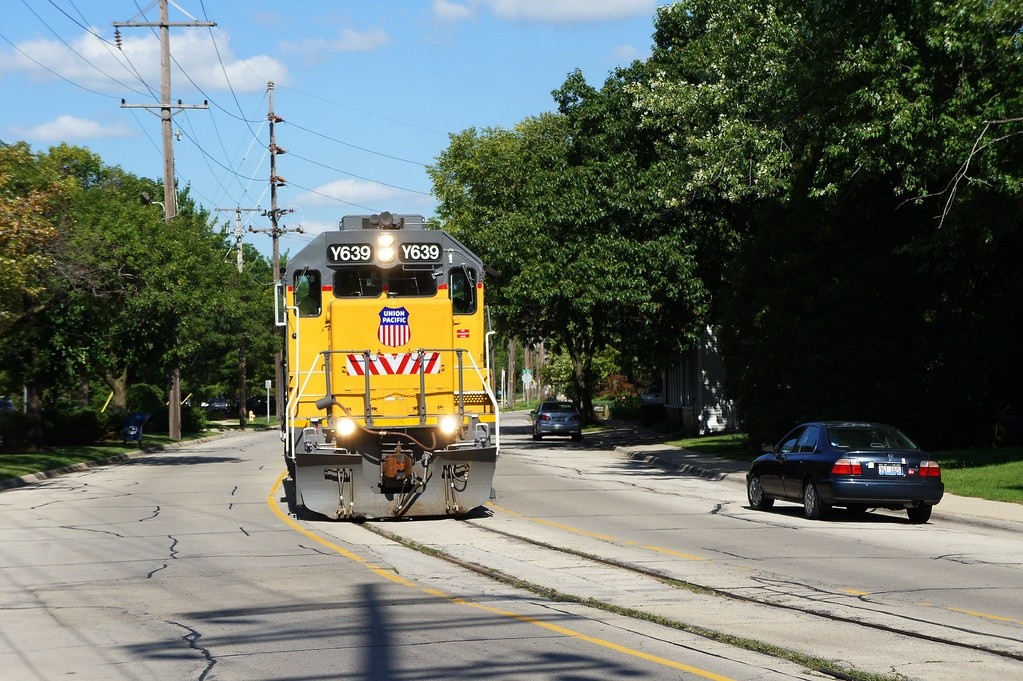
[286,211,500,520]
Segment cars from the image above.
[204,399,234,420]
[746,420,946,523]
[531,400,583,442]
[236,395,276,416]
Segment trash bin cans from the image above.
[122,411,152,447]
